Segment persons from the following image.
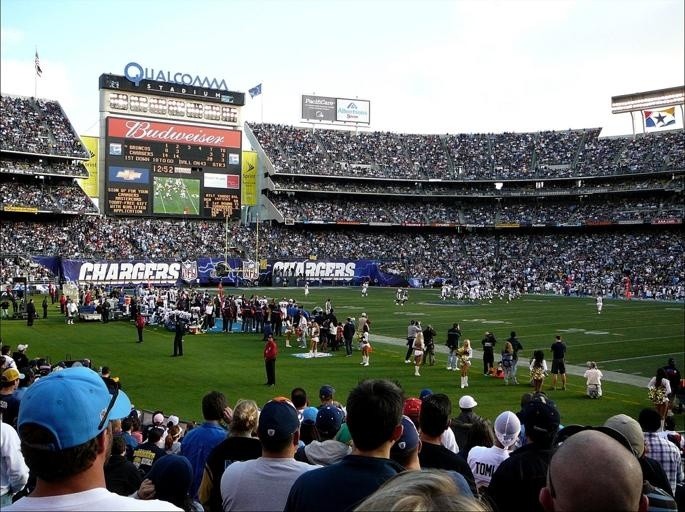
[0,93,684,510]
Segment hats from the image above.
[17,366,131,452]
[259,384,479,453]
[154,413,164,425]
[1,368,26,382]
[145,455,193,499]
[524,396,560,443]
[602,414,644,459]
[18,344,28,351]
[167,415,179,427]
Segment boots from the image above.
[286,340,292,347]
[461,376,468,388]
[360,356,369,366]
[414,366,421,376]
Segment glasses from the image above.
[98,376,119,431]
[547,424,643,505]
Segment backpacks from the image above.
[502,352,514,367]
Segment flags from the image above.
[33,52,42,77]
[248,83,261,99]
